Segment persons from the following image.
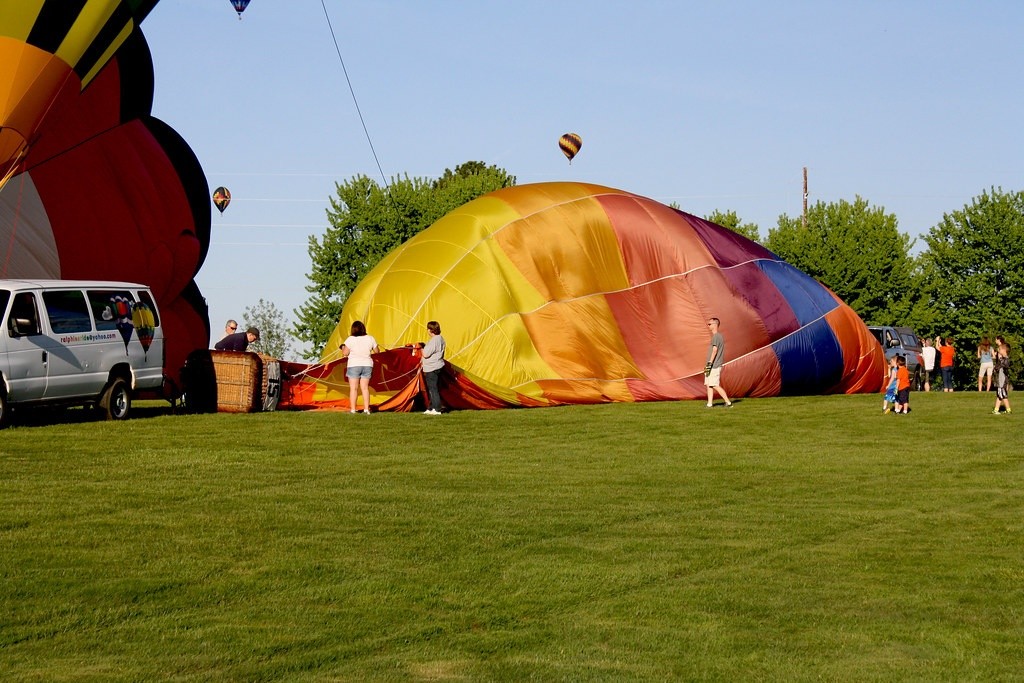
[881,355,910,415]
[703,318,732,408]
[919,336,956,392]
[341,321,379,415]
[214,320,260,352]
[977,335,1015,415]
[421,321,446,415]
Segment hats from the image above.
[248,327,261,341]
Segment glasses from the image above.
[227,324,237,330]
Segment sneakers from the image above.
[424,409,442,415]
[883,408,891,414]
[1004,409,1012,415]
[990,410,1001,414]
[362,410,371,415]
[723,402,733,408]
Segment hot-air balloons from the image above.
[213,187,231,217]
[196,181,889,412]
[229,0,250,21]
[559,133,583,165]
[0,0,211,402]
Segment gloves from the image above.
[703,361,714,376]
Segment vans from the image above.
[0,279,166,422]
[867,325,923,392]
